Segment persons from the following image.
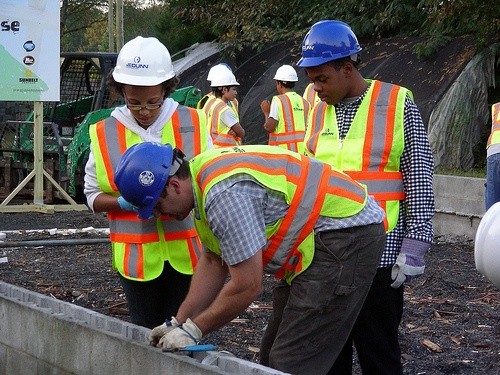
[486,85,500,213]
[84,36,212,331]
[114,142,386,375]
[196,63,245,151]
[296,21,437,375]
[261,64,321,156]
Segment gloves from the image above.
[391,237,431,289]
[148,316,203,354]
[118,195,155,219]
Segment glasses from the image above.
[122,88,164,111]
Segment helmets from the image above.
[113,141,173,219]
[111,36,176,86]
[296,20,362,67]
[273,65,299,81]
[207,64,232,81]
[210,68,240,87]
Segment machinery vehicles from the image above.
[0,40,231,204]
